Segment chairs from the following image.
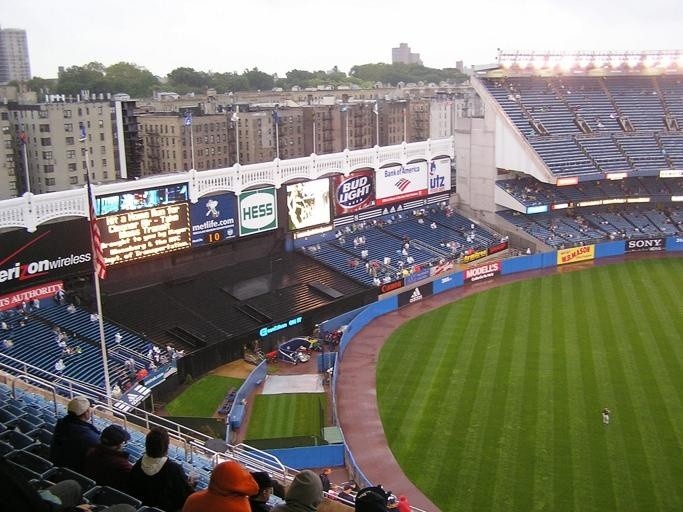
[297,210,497,289]
[480,72,683,247]
[0,303,283,512]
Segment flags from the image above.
[184,113,192,126]
[77,126,86,142]
[18,132,27,145]
[372,103,378,115]
[271,110,280,124]
[312,109,317,123]
[340,106,348,112]
[85,169,106,280]
[230,112,239,122]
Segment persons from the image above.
[489,72,682,257]
[248,471,279,512]
[0,285,185,401]
[0,454,138,512]
[303,198,478,285]
[82,424,134,494]
[125,427,196,512]
[600,408,610,426]
[48,394,103,479]
[179,460,259,512]
[269,466,412,512]
[295,322,343,354]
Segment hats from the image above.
[248,471,279,488]
[354,486,388,511]
[98,424,131,447]
[67,395,92,418]
